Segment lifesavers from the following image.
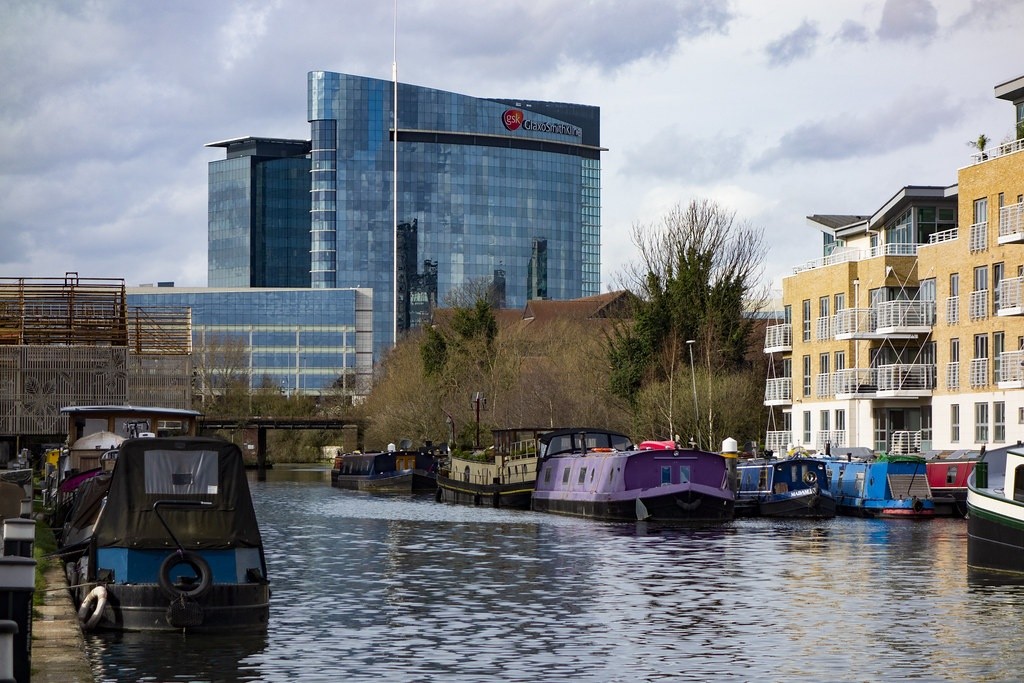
[591,447,617,452]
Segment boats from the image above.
[330,439,453,499]
[965,440,1024,586]
[531,428,737,529]
[814,440,937,519]
[40,404,273,640]
[731,440,837,520]
[436,427,566,508]
[922,449,991,520]
